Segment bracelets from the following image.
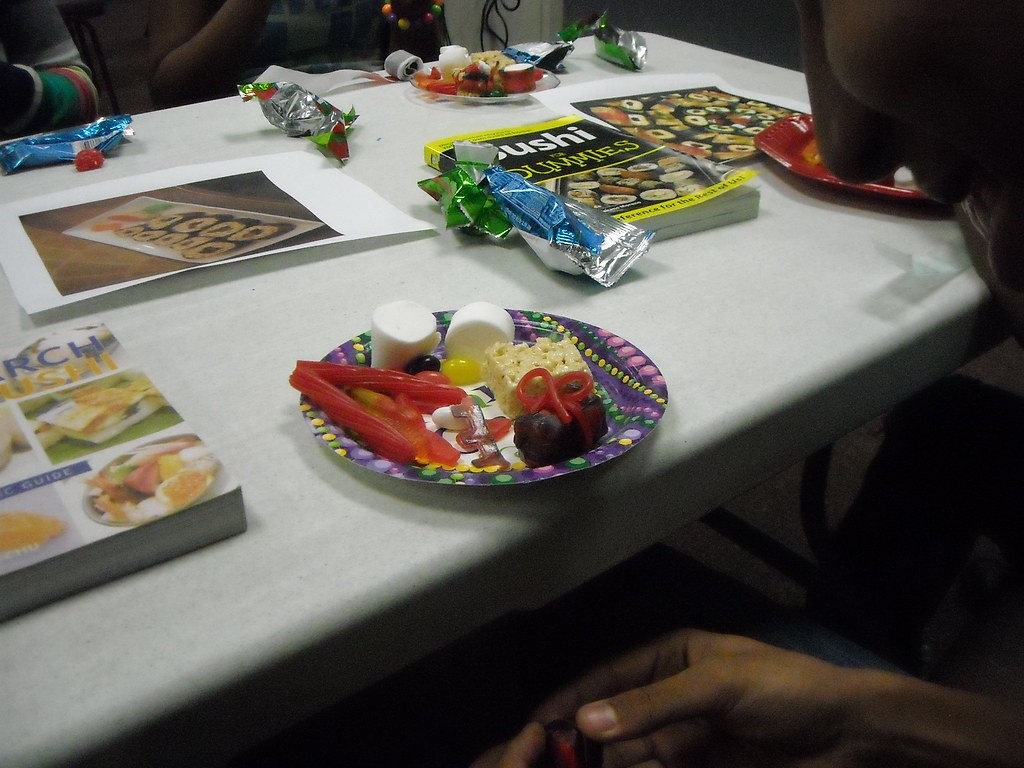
[381,0,444,30]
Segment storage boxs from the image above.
[0,320,246,623]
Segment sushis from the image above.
[116,213,282,260]
[566,157,706,210]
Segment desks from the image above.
[0,30,991,768]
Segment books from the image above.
[424,114,761,244]
[0,322,248,620]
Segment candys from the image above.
[414,60,508,97]
[289,353,514,474]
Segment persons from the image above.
[0,0,98,143]
[473,0,1024,768]
[146,0,444,110]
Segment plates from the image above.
[591,85,790,154]
[753,113,930,200]
[408,68,562,104]
[12,324,221,528]
[299,308,670,486]
[566,163,706,205]
[62,194,326,265]
